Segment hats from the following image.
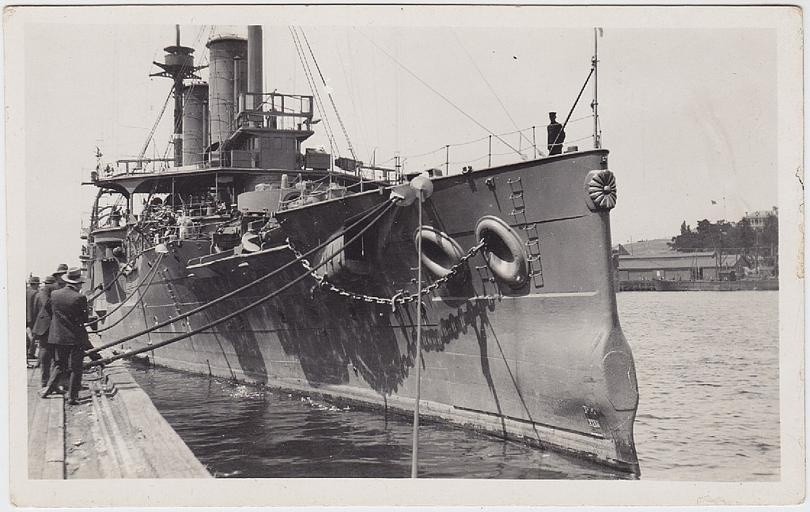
[61,266,87,284]
[29,277,41,284]
[43,275,56,284]
[51,264,68,276]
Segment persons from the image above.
[27,259,88,405]
[546,112,566,157]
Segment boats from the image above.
[77,19,644,476]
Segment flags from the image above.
[710,199,724,206]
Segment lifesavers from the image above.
[475,215,529,289]
[413,226,466,278]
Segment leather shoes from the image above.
[68,399,79,404]
[37,390,47,398]
